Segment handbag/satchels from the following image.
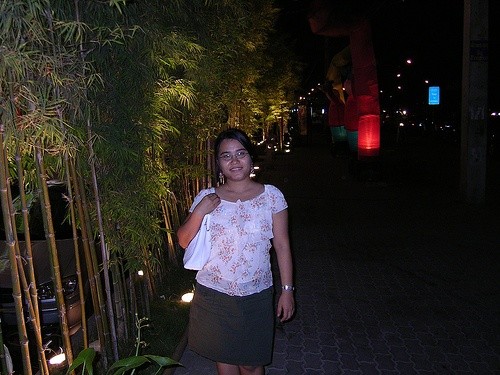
[183,214,211,270]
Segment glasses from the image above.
[217,152,249,161]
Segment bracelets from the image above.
[282,285,294,290]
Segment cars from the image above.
[0,178,103,337]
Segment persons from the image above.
[177,129,296,375]
